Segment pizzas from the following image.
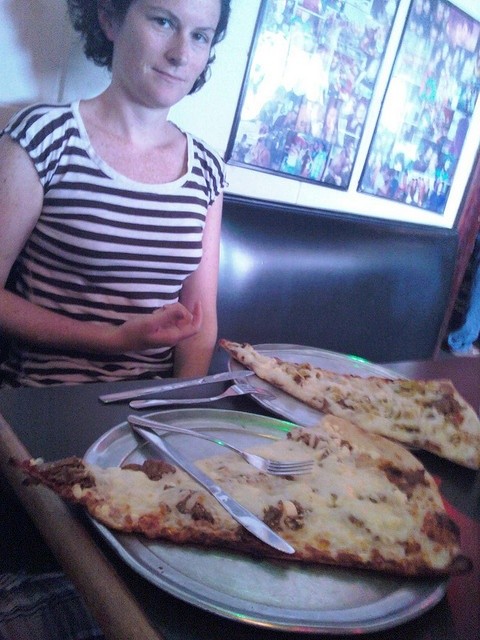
[219,337,480,473]
[8,414,474,579]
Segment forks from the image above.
[128,383,259,409]
[127,414,314,476]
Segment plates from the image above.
[80,407,448,636]
[227,343,422,451]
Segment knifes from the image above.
[131,423,295,555]
[98,370,255,404]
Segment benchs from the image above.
[206,189,472,375]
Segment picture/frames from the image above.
[225,0,396,196]
[353,0,478,217]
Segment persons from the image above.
[358,0,480,215]
[0,0,228,388]
[232,0,398,189]
